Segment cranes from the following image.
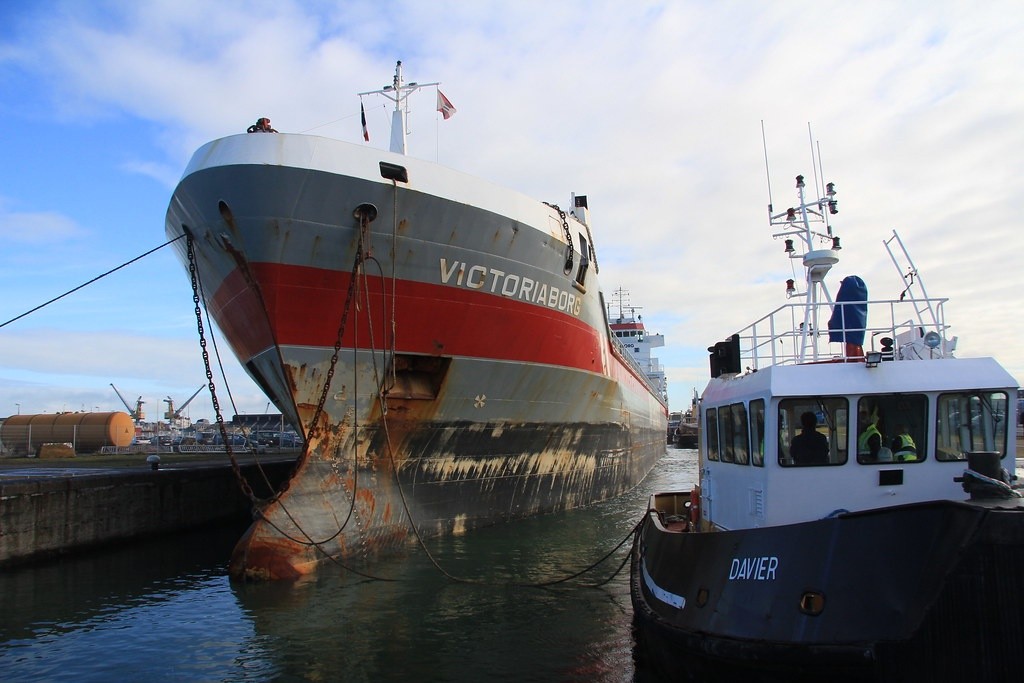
[161,384,207,425]
[110,382,145,426]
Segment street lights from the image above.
[15,402,21,415]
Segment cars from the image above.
[133,436,152,445]
[212,432,257,449]
[196,431,215,445]
[258,431,304,447]
[173,436,207,450]
[153,435,174,446]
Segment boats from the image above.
[627,121,1024,670]
[162,51,672,583]
[673,386,703,447]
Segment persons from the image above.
[790,411,830,468]
[856,408,883,464]
[891,422,918,463]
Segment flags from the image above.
[360,102,369,144]
[437,91,457,121]
